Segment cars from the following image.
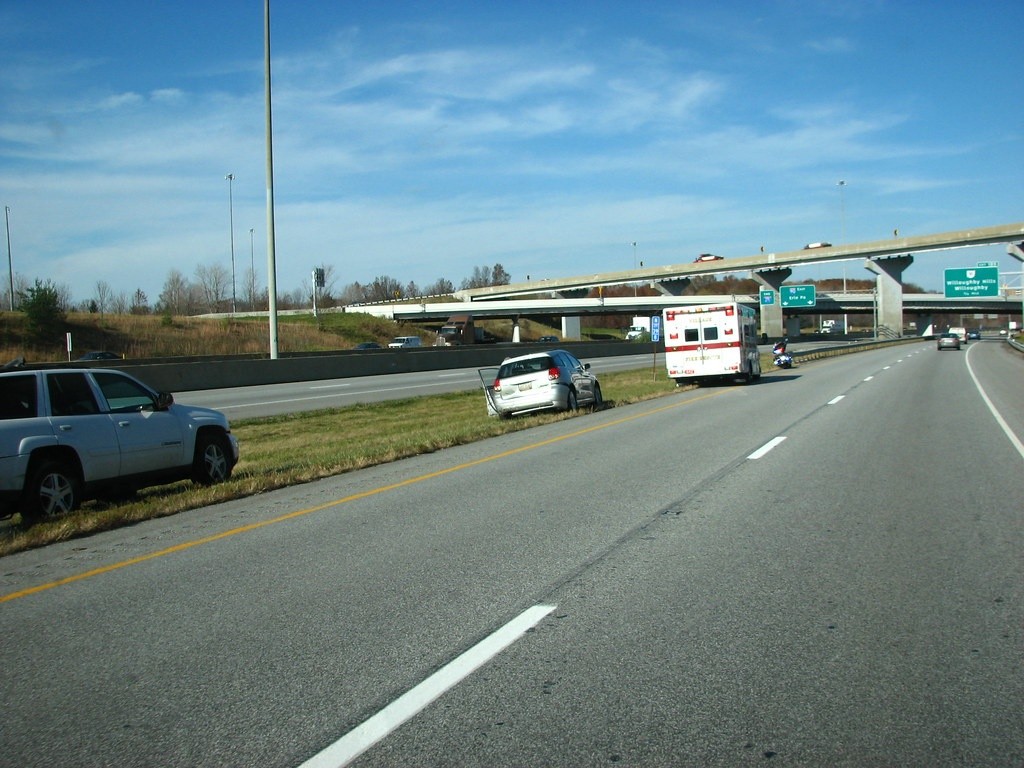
[937,332,960,350]
[538,336,559,342]
[71,351,122,360]
[999,329,1008,335]
[805,241,832,249]
[478,349,604,420]
[967,329,980,340]
[948,326,968,344]
[354,342,381,350]
[435,326,463,340]
[693,253,724,263]
[484,330,496,344]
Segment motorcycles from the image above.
[772,338,793,369]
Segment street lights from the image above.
[631,240,638,270]
[5,205,16,312]
[248,227,256,311]
[223,172,237,312]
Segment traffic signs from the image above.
[945,267,999,298]
[779,285,816,306]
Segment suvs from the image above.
[0,356,239,531]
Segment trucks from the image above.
[817,320,835,328]
[624,316,664,340]
[446,313,483,344]
[387,336,421,348]
[662,302,761,383]
[814,328,830,334]
[861,328,872,333]
